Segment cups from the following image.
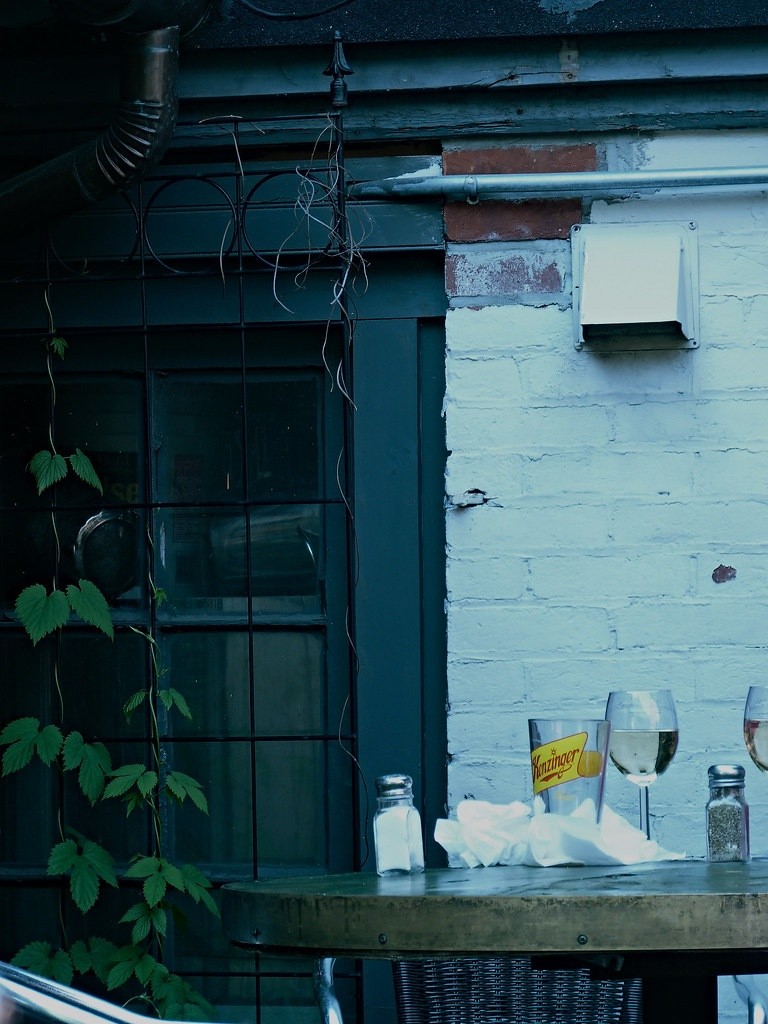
[527,717,610,832]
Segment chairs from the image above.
[392,961,641,1024]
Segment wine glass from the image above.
[606,689,679,842]
[743,685,768,773]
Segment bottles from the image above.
[705,764,751,865]
[372,773,425,877]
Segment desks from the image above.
[220,857,768,1024]
